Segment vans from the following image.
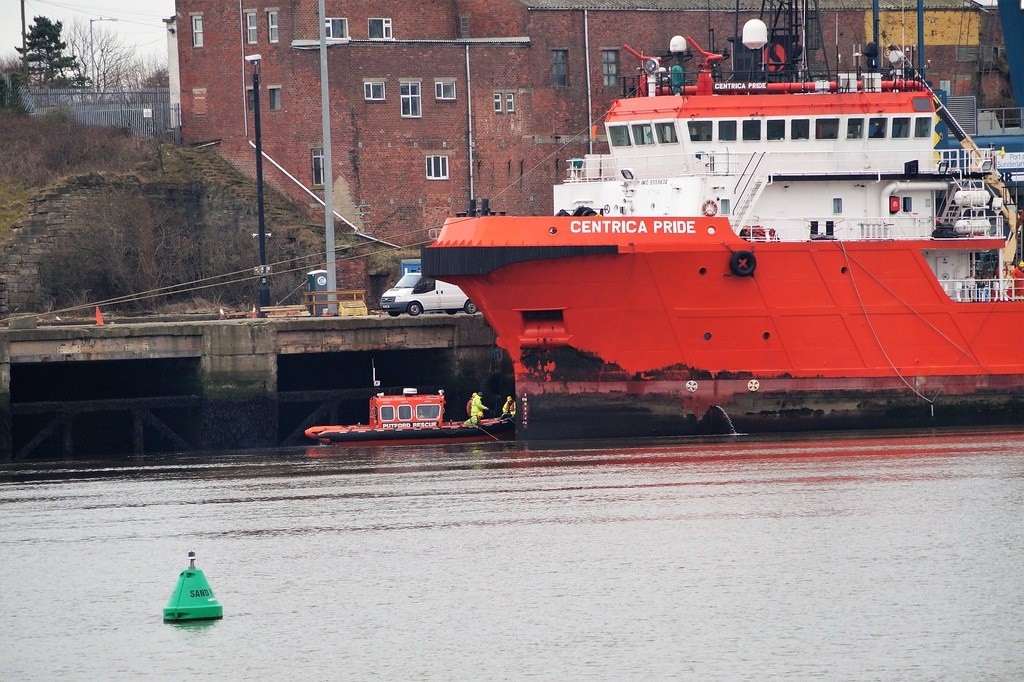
[380,273,478,317]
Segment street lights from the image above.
[245,53,271,318]
[90,18,118,88]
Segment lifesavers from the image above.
[730,250,756,277]
[703,200,718,217]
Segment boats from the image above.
[305,388,517,447]
[422,20,1024,440]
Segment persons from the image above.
[463,392,489,426]
[502,396,516,415]
[418,410,426,420]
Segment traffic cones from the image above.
[95,305,106,326]
[219,306,225,320]
[252,304,257,317]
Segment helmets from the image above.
[507,396,512,401]
[472,393,477,397]
